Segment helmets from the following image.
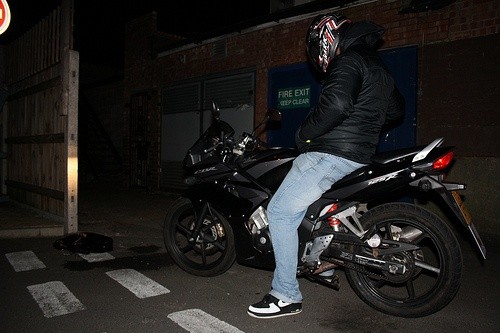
[305,13,353,74]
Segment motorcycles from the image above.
[162,99,488,318]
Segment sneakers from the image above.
[246,294,303,320]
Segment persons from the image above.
[247,13,394,319]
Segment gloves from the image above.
[295,127,305,149]
